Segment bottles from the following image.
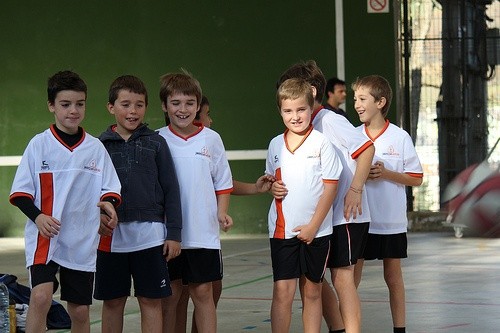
[0,277,10,333]
[9,305,16,333]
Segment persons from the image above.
[154,65,276,333]
[9,69,121,333]
[265,58,424,333]
[93,74,183,333]
